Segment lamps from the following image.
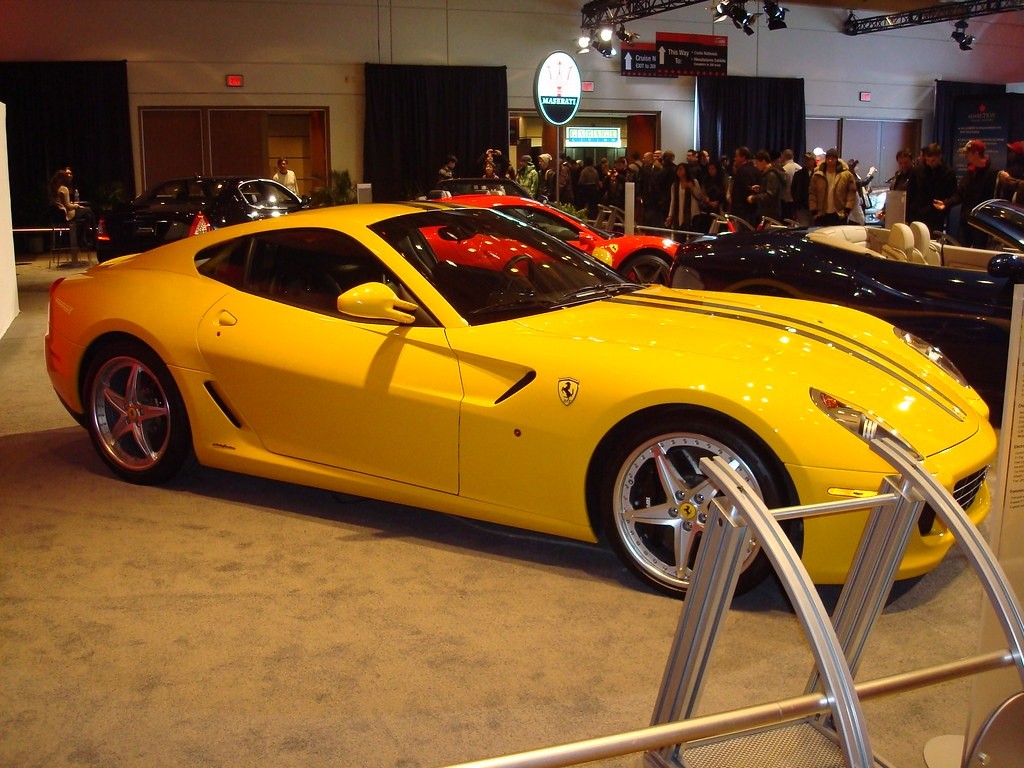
[950,17,976,51]
[711,0,790,32]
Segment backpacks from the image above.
[633,162,653,190]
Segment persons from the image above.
[878,138,1024,246]
[478,148,876,248]
[49,167,97,250]
[271,155,300,204]
[432,156,457,191]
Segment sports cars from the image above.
[46,198,1000,605]
[665,197,1024,391]
[393,191,686,303]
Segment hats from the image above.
[538,153,553,166]
[958,140,986,157]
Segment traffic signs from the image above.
[621,41,677,79]
[655,30,731,76]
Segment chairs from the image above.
[45,201,75,270]
[881,219,946,268]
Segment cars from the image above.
[92,175,314,262]
[408,178,577,239]
